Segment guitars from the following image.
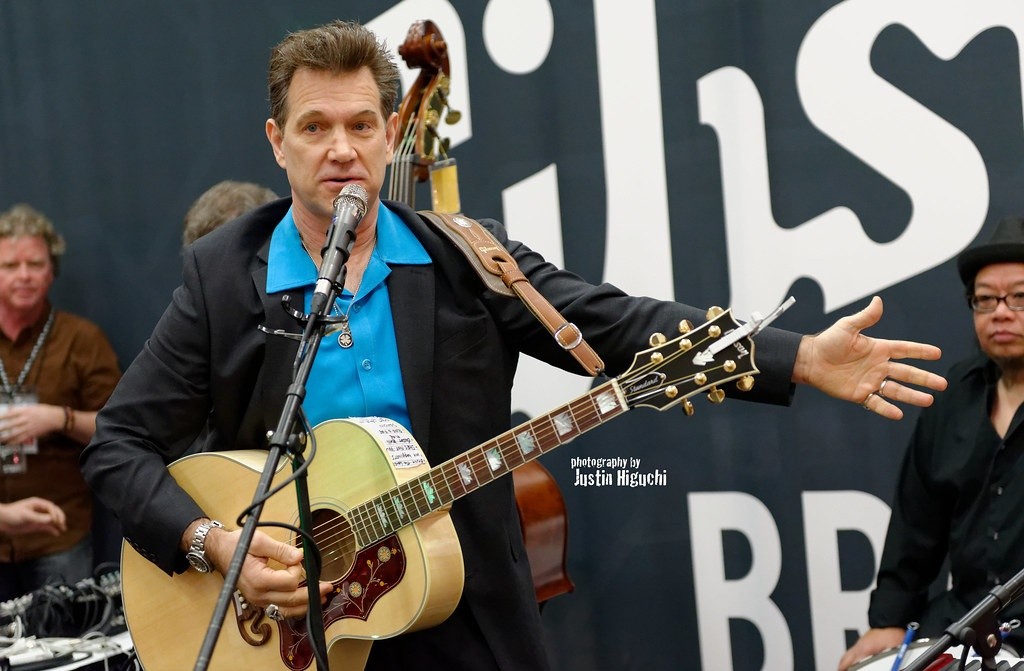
[115,295,808,671]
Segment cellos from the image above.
[383,15,575,607]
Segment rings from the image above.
[265,604,284,622]
[864,393,875,411]
[879,380,888,396]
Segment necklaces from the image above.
[300,238,376,348]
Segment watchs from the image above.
[185,520,227,574]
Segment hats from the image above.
[959,215,1024,290]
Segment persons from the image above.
[839,219,1023,670]
[0,496,67,537]
[180,179,282,247]
[78,19,949,671]
[0,202,121,634]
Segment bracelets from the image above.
[63,405,75,433]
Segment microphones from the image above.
[309,183,369,316]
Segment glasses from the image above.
[972,292,1024,312]
